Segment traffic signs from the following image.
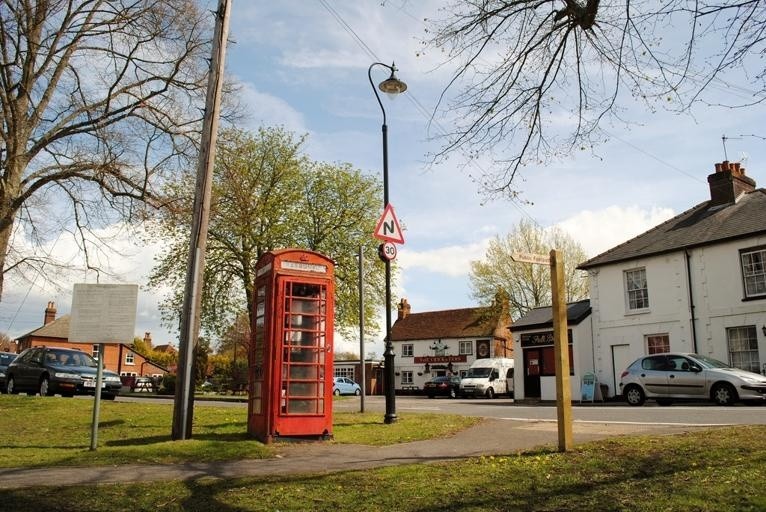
[383,242,397,260]
[510,250,552,266]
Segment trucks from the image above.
[458,358,513,398]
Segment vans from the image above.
[506,368,514,395]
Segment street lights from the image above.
[366,59,407,423]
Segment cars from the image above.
[424,376,462,398]
[332,376,362,395]
[5,344,122,400]
[0,351,19,393]
[618,352,766,405]
